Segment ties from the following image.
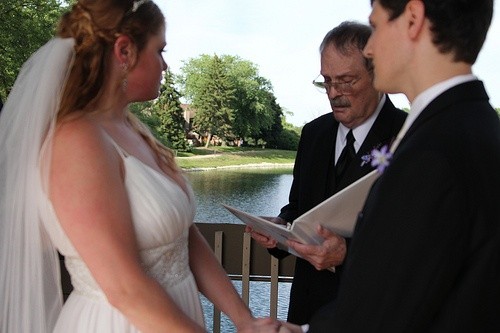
[333,130,359,191]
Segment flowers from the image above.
[370,146,393,173]
[361,155,371,167]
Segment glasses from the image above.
[312,69,372,95]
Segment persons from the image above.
[0,0,282,333]
[267,0,500,333]
[245,22,408,333]
